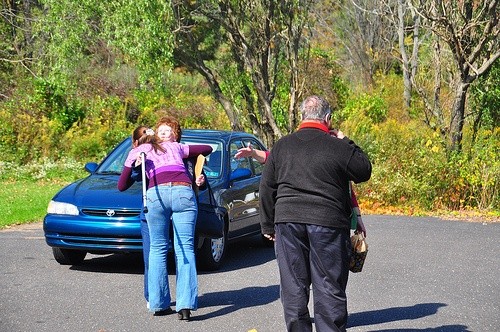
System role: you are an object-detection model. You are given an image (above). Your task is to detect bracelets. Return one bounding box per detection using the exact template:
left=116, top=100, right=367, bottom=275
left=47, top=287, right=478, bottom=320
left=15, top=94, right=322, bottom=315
left=357, top=215, right=361, bottom=217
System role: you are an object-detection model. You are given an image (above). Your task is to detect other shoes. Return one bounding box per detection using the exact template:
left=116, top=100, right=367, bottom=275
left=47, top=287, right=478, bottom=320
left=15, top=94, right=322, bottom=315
left=179, top=309, right=191, bottom=319
left=154, top=309, right=177, bottom=316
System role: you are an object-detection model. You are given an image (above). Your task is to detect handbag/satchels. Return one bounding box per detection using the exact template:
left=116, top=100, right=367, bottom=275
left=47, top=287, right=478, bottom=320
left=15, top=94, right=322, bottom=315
left=194, top=181, right=228, bottom=239
left=349, top=233, right=369, bottom=273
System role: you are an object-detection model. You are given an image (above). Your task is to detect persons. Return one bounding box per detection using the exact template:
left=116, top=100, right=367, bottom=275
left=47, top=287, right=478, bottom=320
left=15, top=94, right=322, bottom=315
left=118, top=125, right=213, bottom=320
left=234, top=94, right=372, bottom=332
left=139, top=117, right=208, bottom=309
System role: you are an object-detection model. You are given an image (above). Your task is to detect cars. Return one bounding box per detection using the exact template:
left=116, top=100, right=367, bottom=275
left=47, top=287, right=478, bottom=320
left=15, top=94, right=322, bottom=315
left=42, top=129, right=274, bottom=271
